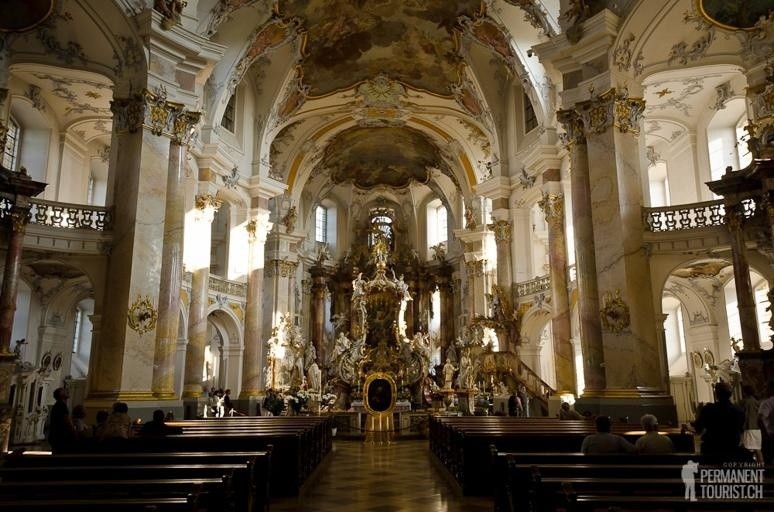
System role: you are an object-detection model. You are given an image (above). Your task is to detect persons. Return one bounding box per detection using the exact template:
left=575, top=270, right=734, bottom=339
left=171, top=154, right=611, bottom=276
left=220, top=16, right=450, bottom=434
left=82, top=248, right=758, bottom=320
left=289, top=332, right=475, bottom=390
left=43, top=380, right=774, bottom=458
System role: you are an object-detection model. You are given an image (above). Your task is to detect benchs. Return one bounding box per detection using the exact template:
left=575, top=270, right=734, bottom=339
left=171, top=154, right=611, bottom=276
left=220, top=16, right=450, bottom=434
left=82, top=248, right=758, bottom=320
left=428, top=408, right=774, bottom=512
left=2, top=411, right=335, bottom=512
